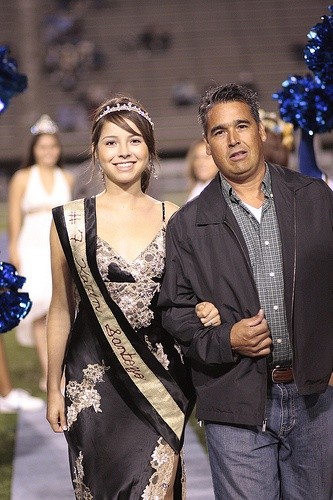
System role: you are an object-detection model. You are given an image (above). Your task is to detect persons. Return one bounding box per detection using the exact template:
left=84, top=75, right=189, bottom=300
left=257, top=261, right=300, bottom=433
left=0, top=335, right=44, bottom=413
left=185, top=139, right=218, bottom=204
left=46, top=97, right=221, bottom=500
left=157, top=83, right=333, bottom=500
left=7, top=114, right=74, bottom=392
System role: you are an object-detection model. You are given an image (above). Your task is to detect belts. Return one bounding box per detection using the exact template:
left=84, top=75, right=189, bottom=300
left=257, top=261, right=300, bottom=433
left=267, top=366, right=294, bottom=383
